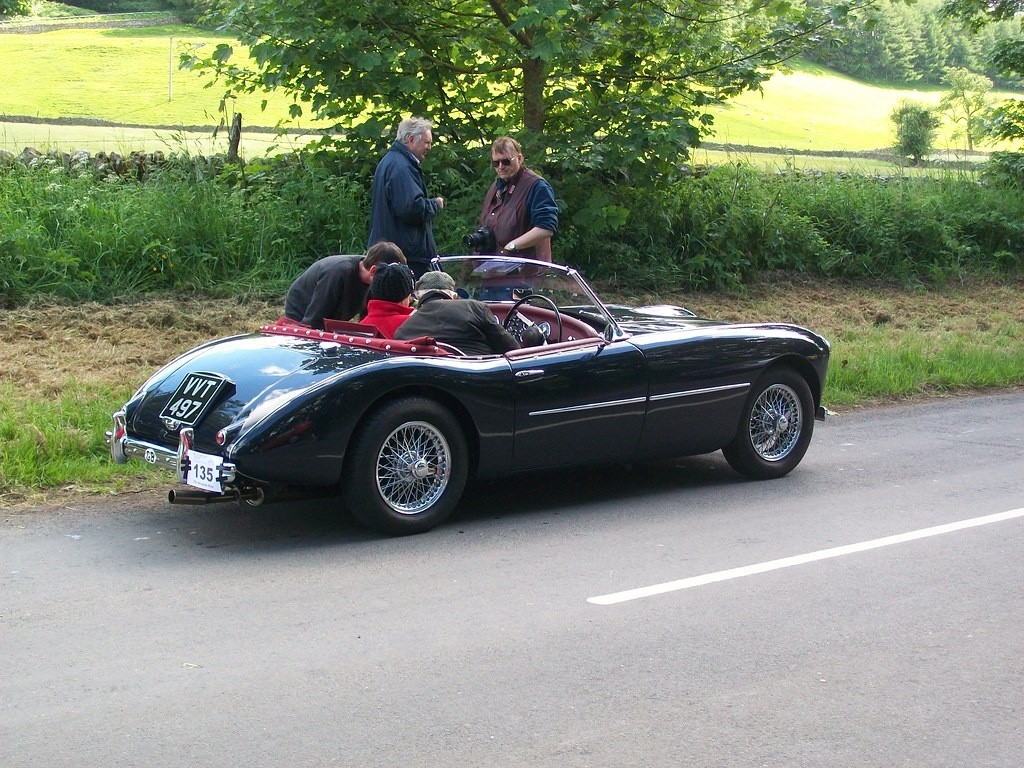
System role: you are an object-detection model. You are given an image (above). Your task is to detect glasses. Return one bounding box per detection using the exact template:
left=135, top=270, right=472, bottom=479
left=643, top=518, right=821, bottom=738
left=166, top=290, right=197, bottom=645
left=492, top=155, right=517, bottom=168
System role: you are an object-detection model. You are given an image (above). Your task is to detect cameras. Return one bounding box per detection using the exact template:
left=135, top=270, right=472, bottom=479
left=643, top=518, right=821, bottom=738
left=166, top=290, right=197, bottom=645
left=463, top=224, right=496, bottom=254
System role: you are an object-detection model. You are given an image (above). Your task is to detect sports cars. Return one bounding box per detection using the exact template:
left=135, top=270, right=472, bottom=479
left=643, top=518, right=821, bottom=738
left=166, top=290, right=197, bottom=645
left=101, top=254, right=832, bottom=536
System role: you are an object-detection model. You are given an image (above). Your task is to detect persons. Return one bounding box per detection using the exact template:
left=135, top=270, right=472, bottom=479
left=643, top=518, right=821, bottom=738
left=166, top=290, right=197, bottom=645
left=367, top=117, right=444, bottom=281
left=358, top=263, right=520, bottom=356
left=285, top=242, right=407, bottom=331
left=472, top=136, right=558, bottom=305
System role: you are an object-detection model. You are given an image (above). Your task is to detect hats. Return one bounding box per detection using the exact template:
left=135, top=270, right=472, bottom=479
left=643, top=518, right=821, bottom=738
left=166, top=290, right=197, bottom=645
left=414, top=270, right=456, bottom=290
left=371, top=262, right=415, bottom=302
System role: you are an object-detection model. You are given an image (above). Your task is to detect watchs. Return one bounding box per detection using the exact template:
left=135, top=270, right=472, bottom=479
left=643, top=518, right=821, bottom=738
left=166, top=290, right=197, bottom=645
left=509, top=242, right=519, bottom=252
